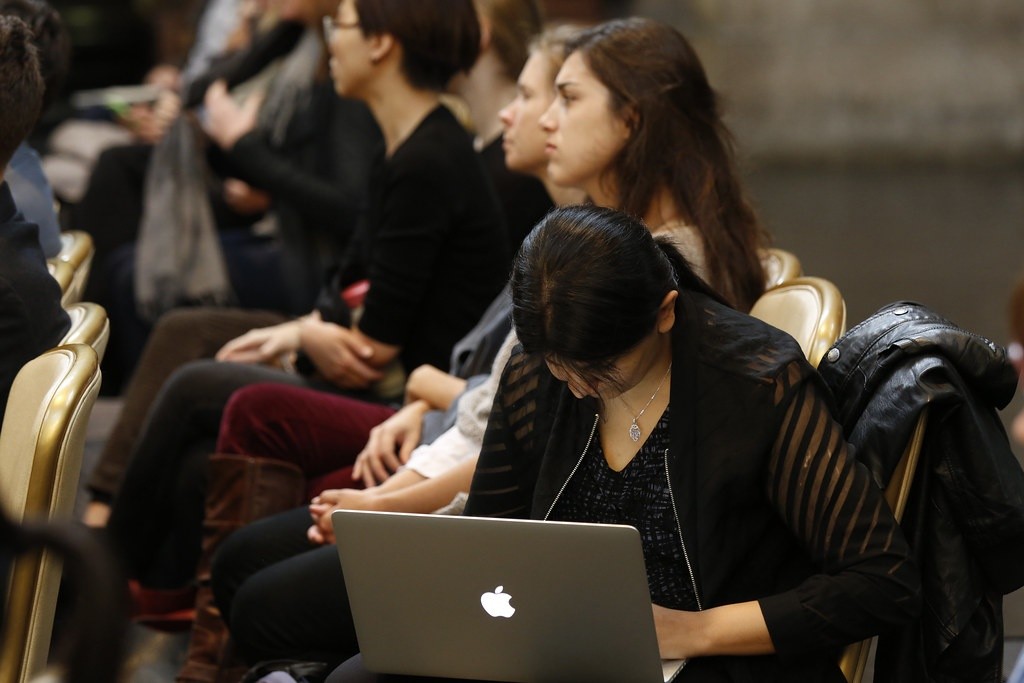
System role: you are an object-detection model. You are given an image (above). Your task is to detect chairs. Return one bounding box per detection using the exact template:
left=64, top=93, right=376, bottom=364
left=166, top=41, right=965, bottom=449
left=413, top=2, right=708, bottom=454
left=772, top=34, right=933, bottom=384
left=0, top=220, right=110, bottom=681
left=736, top=245, right=927, bottom=682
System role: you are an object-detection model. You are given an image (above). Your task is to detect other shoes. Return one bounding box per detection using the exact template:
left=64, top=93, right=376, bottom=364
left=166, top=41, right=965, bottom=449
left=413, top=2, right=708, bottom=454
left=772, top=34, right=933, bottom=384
left=133, top=592, right=199, bottom=624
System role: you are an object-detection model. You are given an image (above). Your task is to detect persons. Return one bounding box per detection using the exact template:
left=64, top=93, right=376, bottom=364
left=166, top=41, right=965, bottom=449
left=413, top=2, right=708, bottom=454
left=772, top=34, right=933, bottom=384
left=325, top=204, right=911, bottom=683
left=0, top=0, right=765, bottom=683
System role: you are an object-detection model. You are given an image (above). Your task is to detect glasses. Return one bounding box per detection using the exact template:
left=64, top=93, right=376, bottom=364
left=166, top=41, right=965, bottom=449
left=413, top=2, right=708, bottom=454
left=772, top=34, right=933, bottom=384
left=322, top=14, right=361, bottom=47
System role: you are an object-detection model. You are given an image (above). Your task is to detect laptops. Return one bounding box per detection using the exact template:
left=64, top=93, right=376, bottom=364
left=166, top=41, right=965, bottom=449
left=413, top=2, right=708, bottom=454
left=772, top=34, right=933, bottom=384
left=331, top=508, right=688, bottom=683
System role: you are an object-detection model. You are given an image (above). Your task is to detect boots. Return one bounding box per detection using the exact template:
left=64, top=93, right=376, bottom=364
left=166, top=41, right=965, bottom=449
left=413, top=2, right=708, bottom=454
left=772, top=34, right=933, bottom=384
left=176, top=454, right=301, bottom=683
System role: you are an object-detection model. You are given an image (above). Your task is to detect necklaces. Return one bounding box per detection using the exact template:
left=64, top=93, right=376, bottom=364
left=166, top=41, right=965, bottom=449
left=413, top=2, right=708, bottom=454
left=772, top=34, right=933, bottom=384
left=626, top=363, right=671, bottom=442
